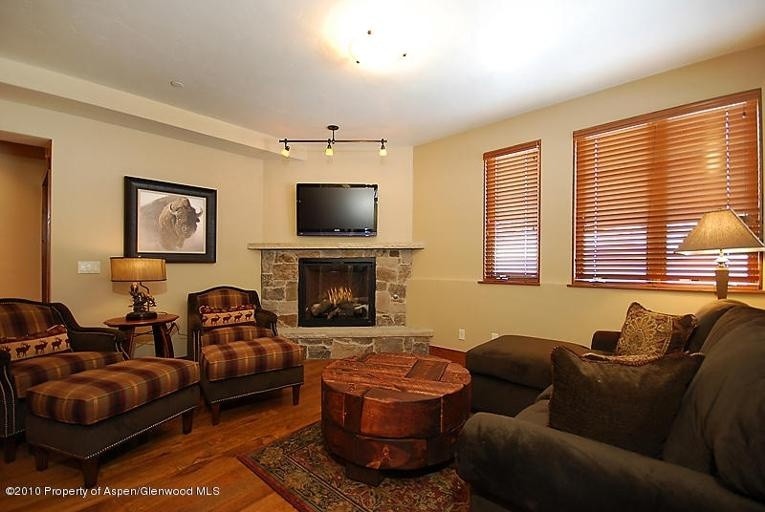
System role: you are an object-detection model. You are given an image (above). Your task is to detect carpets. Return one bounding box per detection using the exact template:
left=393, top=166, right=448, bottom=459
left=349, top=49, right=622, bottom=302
left=237, top=419, right=470, bottom=512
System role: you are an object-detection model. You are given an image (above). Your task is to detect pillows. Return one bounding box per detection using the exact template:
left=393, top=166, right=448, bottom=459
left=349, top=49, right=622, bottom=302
left=614, top=302, right=699, bottom=357
left=549, top=344, right=706, bottom=461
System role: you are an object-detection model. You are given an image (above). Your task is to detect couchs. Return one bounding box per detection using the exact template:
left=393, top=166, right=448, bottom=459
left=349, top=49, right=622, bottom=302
left=456, top=299, right=765, bottom=512
left=187, top=285, right=305, bottom=426
left=0, top=298, right=132, bottom=463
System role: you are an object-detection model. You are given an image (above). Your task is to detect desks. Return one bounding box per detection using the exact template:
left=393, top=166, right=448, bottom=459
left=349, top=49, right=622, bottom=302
left=103, top=312, right=180, bottom=359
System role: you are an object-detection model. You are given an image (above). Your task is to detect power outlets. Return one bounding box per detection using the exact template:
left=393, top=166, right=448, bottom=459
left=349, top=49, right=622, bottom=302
left=76, top=261, right=101, bottom=274
left=458, top=329, right=466, bottom=339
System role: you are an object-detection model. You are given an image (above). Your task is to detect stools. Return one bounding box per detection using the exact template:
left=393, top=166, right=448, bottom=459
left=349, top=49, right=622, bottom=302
left=25, top=356, right=200, bottom=489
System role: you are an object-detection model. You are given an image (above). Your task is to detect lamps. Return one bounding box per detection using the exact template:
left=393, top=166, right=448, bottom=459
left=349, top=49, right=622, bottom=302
left=338, top=10, right=421, bottom=83
left=279, top=124, right=388, bottom=158
left=110, top=256, right=167, bottom=321
left=673, top=208, right=765, bottom=300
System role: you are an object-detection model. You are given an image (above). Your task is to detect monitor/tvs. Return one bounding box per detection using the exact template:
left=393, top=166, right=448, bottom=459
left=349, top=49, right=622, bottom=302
left=296, top=183, right=378, bottom=237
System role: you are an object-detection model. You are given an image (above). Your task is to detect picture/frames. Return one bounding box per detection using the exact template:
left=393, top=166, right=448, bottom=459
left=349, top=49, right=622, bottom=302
left=123, top=175, right=217, bottom=264
